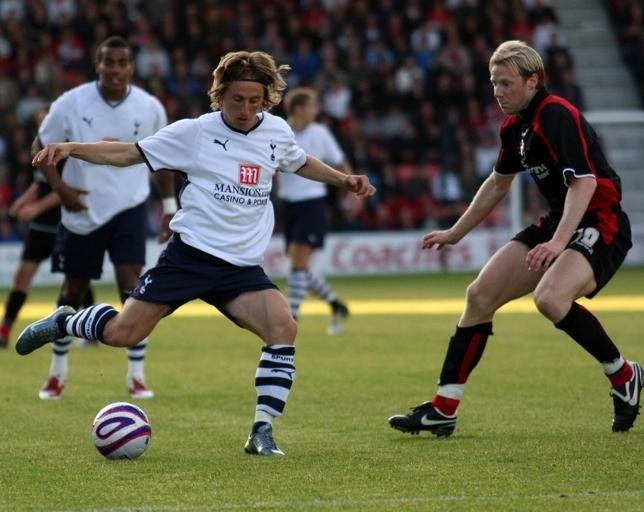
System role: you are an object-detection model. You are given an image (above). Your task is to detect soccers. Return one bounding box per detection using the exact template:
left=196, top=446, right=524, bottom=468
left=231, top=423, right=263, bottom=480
left=91, top=402, right=151, bottom=460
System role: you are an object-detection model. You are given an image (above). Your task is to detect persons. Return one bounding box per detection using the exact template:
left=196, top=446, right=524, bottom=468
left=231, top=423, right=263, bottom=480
left=31, top=37, right=177, bottom=401
left=0, top=106, right=96, bottom=346
left=15, top=50, right=377, bottom=455
left=1, top=4, right=644, bottom=241
left=273, top=87, right=348, bottom=335
left=389, top=40, right=643, bottom=437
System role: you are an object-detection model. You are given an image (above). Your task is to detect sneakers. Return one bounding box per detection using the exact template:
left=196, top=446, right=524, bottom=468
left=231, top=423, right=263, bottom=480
left=611, top=363, right=644, bottom=433
left=389, top=401, right=458, bottom=437
left=40, top=374, right=67, bottom=401
left=326, top=306, right=350, bottom=336
left=14, top=304, right=76, bottom=356
left=245, top=424, right=284, bottom=457
left=126, top=376, right=153, bottom=399
